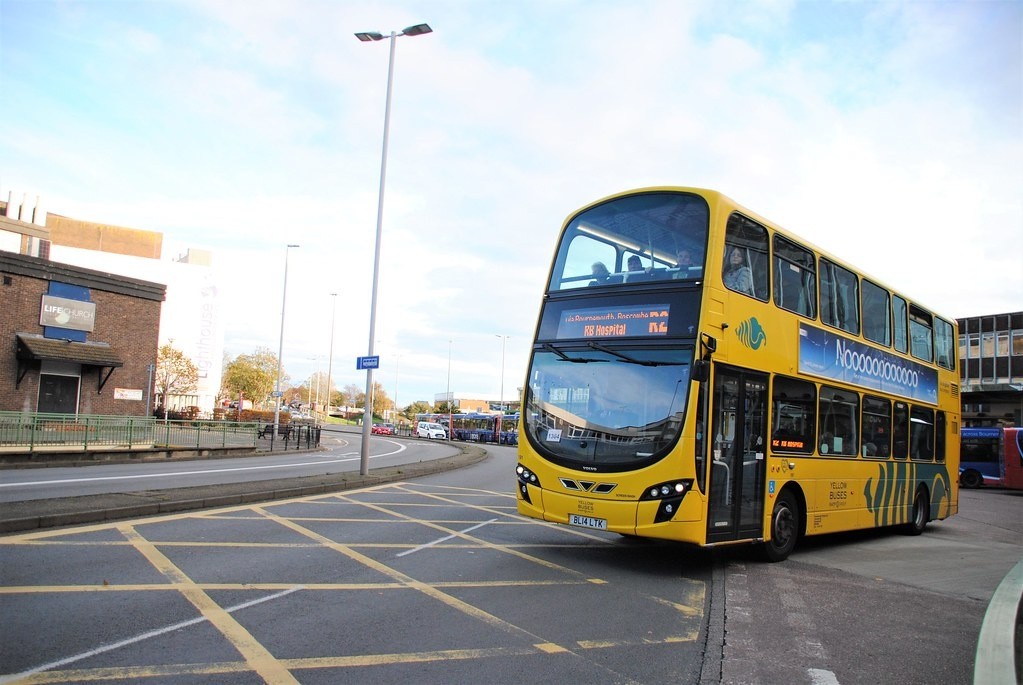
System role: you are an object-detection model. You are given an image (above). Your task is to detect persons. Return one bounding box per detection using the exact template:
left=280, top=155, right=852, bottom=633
left=938, top=356, right=946, bottom=366
left=724, top=245, right=753, bottom=297
left=822, top=428, right=891, bottom=456
left=580, top=249, right=698, bottom=287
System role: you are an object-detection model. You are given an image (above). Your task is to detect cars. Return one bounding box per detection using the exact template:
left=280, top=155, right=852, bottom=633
left=222, top=399, right=239, bottom=408
left=280, top=398, right=308, bottom=420
left=382, top=423, right=399, bottom=435
left=372, top=423, right=391, bottom=435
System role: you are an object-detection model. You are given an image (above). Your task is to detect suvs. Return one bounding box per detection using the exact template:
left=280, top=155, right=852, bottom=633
left=417, top=421, right=447, bottom=440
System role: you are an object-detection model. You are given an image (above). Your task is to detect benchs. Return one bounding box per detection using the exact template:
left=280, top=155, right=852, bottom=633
left=258, top=425, right=292, bottom=441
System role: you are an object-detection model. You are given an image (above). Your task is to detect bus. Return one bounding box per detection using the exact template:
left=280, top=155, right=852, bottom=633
left=958, top=426, right=1023, bottom=491
left=412, top=412, right=538, bottom=446
left=514, top=183, right=962, bottom=565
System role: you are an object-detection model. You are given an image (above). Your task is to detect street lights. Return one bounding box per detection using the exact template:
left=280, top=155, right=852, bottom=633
left=325, top=292, right=342, bottom=417
left=352, top=21, right=436, bottom=478
left=495, top=333, right=510, bottom=446
left=273, top=243, right=302, bottom=442
left=164, top=338, right=177, bottom=425
